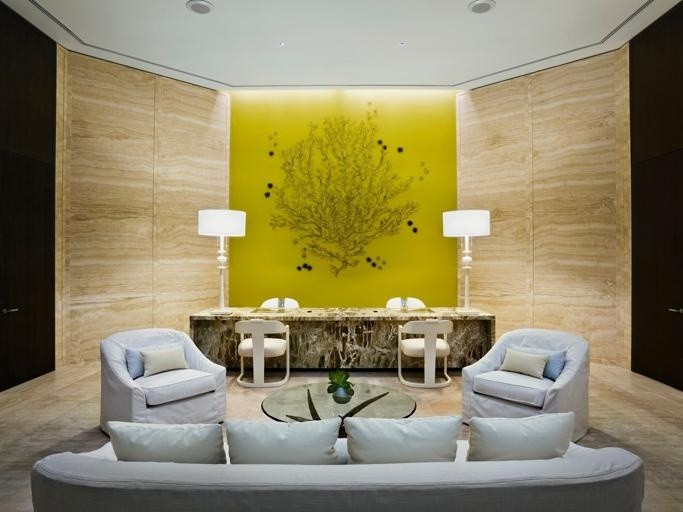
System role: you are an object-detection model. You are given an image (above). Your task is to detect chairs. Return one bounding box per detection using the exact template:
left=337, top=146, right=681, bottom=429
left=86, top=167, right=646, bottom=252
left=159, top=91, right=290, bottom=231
left=260, top=298, right=300, bottom=310
left=386, top=298, right=426, bottom=311
left=398, top=320, right=451, bottom=388
left=235, top=321, right=290, bottom=387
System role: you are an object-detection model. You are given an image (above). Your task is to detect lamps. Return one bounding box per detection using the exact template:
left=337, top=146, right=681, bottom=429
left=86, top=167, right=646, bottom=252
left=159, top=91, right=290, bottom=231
left=443, top=209, right=490, bottom=315
left=198, top=210, right=245, bottom=315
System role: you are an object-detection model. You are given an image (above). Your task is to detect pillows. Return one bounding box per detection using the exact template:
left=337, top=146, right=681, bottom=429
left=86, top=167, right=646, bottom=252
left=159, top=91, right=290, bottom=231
left=124, top=349, right=144, bottom=380
left=467, top=411, right=576, bottom=462
left=498, top=350, right=547, bottom=380
left=106, top=420, right=226, bottom=465
left=229, top=419, right=340, bottom=464
left=343, top=417, right=459, bottom=463
left=141, top=345, right=188, bottom=377
left=509, top=345, right=567, bottom=380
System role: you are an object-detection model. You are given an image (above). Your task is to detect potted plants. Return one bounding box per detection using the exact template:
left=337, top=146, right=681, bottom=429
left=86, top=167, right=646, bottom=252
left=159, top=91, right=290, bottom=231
left=327, top=372, right=354, bottom=403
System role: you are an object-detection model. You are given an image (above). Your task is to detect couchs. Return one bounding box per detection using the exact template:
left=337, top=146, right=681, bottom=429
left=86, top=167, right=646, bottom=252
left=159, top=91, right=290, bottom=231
left=461, top=329, right=590, bottom=442
left=31, top=441, right=643, bottom=512
left=101, top=329, right=227, bottom=435
